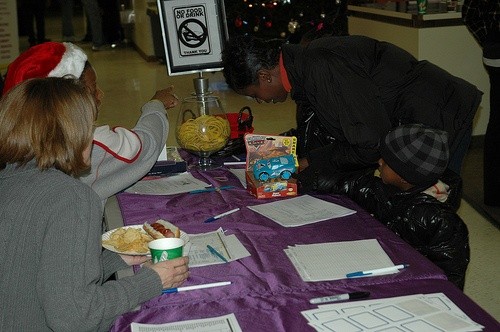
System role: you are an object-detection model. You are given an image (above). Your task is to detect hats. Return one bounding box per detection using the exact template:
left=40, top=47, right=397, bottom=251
left=3, top=42, right=88, bottom=96
left=379, top=123, right=450, bottom=189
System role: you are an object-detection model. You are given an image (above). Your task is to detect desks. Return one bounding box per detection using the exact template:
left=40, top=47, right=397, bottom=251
left=105, top=148, right=499, bottom=332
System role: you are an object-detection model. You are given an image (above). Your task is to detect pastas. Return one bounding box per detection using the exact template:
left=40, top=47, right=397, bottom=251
left=175, top=115, right=231, bottom=151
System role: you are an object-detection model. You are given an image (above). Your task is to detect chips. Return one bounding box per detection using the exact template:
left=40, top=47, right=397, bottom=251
left=101, top=227, right=152, bottom=253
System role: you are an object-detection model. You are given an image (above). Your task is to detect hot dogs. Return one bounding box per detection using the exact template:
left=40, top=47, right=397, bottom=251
left=143, top=220, right=181, bottom=240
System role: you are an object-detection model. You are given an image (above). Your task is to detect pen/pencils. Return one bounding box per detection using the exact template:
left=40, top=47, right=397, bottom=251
left=206, top=244, right=229, bottom=263
left=161, top=281, right=234, bottom=293
left=310, top=291, right=373, bottom=304
left=205, top=206, right=243, bottom=222
left=190, top=186, right=235, bottom=193
left=346, top=263, right=410, bottom=277
left=222, top=162, right=247, bottom=165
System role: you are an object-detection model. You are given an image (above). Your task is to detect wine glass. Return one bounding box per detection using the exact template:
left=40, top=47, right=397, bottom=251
left=175, top=95, right=233, bottom=171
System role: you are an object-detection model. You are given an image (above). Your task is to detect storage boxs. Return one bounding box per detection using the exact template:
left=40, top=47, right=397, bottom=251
left=244, top=133, right=298, bottom=199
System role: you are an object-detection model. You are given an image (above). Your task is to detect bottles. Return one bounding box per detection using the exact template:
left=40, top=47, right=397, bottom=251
left=417, top=0, right=428, bottom=14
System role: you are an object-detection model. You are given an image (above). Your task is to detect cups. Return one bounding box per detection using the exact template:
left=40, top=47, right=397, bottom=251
left=148, top=238, right=184, bottom=264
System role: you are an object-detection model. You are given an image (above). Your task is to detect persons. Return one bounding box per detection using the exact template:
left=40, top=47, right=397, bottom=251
left=295, top=123, right=470, bottom=290
left=29, top=0, right=125, bottom=50
left=222, top=34, right=483, bottom=208
left=463, top=0, right=500, bottom=207
left=0, top=41, right=177, bottom=208
left=0, top=77, right=192, bottom=332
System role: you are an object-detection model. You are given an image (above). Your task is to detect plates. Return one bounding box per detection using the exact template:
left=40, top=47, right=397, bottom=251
left=101, top=225, right=189, bottom=255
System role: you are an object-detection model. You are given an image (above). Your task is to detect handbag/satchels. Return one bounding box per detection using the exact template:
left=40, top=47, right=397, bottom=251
left=182, top=106, right=254, bottom=140
